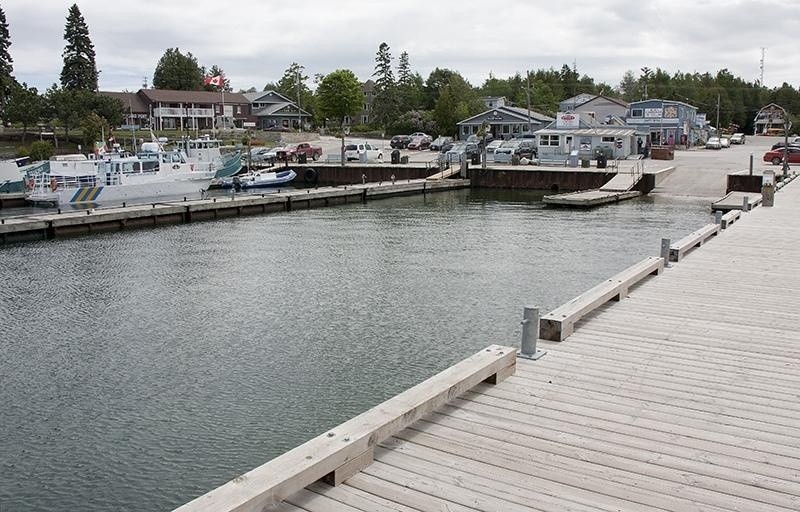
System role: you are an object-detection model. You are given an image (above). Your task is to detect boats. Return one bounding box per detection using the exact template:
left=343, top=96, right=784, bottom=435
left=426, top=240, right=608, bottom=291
left=0, top=97, right=298, bottom=207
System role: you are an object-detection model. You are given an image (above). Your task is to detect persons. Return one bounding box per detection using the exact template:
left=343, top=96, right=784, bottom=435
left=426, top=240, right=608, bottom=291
left=637, top=137, right=643, bottom=153
left=668, top=135, right=673, bottom=144
left=781, top=151, right=792, bottom=170
left=51, top=178, right=58, bottom=192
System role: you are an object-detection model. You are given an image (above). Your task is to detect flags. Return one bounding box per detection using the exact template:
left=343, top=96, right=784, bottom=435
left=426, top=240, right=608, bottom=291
left=204, top=75, right=224, bottom=88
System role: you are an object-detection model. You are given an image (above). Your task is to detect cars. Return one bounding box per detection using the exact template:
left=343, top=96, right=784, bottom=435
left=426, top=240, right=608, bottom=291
left=705, top=133, right=746, bottom=150
left=344, top=143, right=384, bottom=161
left=390, top=132, right=538, bottom=160
left=239, top=142, right=323, bottom=164
left=763, top=136, right=800, bottom=165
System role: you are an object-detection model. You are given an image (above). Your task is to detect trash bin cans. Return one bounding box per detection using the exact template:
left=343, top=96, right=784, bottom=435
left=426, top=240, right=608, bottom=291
left=391, top=150, right=400, bottom=164
left=298, top=152, right=306, bottom=164
left=471, top=149, right=480, bottom=165
left=597, top=152, right=607, bottom=168
